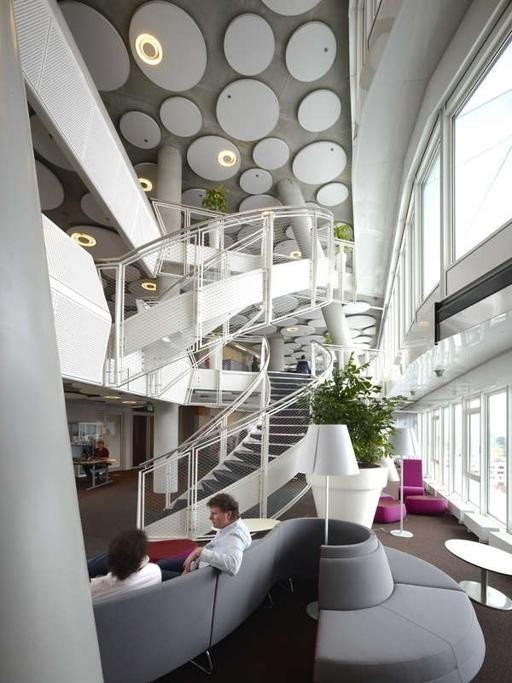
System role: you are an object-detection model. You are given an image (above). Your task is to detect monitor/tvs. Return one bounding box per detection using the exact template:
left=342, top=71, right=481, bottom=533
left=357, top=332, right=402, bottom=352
left=81, top=444, right=95, bottom=458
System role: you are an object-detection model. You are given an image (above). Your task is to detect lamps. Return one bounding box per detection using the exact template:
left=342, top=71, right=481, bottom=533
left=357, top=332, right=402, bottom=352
left=296, top=422, right=360, bottom=545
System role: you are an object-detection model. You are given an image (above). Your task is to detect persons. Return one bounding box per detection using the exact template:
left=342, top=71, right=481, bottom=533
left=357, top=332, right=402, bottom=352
left=89, top=528, right=162, bottom=604
left=156, top=492, right=254, bottom=586
left=82, top=440, right=109, bottom=484
left=295, top=354, right=311, bottom=373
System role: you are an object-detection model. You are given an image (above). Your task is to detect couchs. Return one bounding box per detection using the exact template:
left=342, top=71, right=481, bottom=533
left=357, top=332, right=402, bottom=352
left=94, top=517, right=486, bottom=682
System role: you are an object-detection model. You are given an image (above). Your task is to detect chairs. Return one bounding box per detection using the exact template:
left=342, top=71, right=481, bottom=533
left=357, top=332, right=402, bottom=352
left=399, top=458, right=424, bottom=502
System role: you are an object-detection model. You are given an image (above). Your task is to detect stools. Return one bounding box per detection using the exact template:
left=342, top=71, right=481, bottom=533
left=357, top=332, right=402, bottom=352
left=374, top=495, right=446, bottom=522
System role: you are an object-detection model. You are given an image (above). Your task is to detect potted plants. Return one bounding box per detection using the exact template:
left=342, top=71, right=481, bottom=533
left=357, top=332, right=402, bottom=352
left=294, top=367, right=409, bottom=526
left=200, top=182, right=229, bottom=249
left=335, top=223, right=353, bottom=271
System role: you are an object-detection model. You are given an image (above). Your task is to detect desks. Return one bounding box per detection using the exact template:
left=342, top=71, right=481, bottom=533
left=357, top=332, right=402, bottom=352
left=73, top=458, right=114, bottom=491
